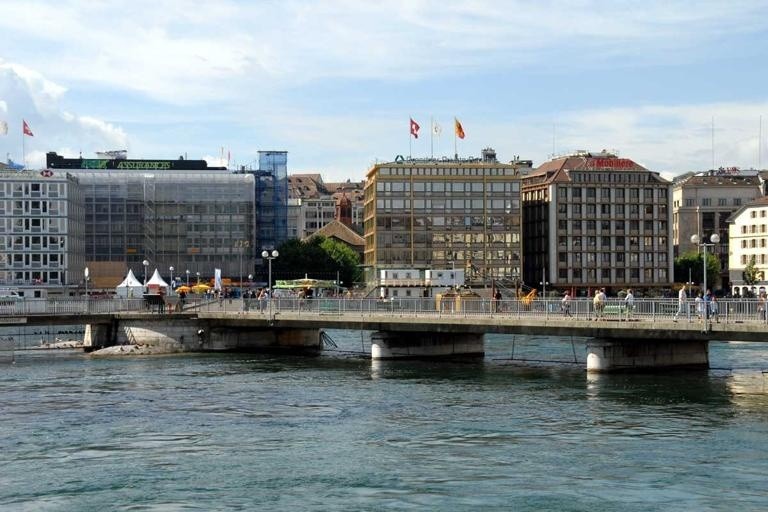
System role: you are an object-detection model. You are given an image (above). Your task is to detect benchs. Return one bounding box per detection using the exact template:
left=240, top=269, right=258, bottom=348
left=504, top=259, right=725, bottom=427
left=662, top=304, right=696, bottom=318
left=377, top=301, right=399, bottom=312
left=604, top=305, right=635, bottom=318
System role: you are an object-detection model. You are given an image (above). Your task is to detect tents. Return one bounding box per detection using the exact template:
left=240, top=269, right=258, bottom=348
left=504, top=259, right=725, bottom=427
left=117, top=268, right=169, bottom=288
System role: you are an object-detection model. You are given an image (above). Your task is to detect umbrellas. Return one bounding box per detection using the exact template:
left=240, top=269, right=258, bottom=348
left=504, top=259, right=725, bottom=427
left=177, top=284, right=210, bottom=294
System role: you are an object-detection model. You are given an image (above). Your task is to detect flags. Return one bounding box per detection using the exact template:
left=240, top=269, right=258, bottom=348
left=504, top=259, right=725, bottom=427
left=410, top=119, right=419, bottom=138
left=23, top=119, right=34, bottom=137
left=455, top=119, right=465, bottom=140
left=432, top=120, right=442, bottom=135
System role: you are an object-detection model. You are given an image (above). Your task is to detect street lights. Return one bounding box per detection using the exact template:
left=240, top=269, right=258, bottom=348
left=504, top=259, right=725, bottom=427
left=447, top=261, right=456, bottom=287
left=539, top=281, right=549, bottom=300
left=261, top=249, right=279, bottom=328
left=84, top=265, right=91, bottom=299
left=689, top=233, right=723, bottom=333
left=332, top=280, right=343, bottom=297
left=686, top=281, right=695, bottom=297
left=247, top=273, right=255, bottom=291
left=142, top=259, right=201, bottom=298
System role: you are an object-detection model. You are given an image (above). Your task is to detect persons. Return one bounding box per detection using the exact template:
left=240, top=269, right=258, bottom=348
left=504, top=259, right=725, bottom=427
left=617, top=288, right=634, bottom=306
left=757, top=292, right=768, bottom=324
left=180, top=290, right=186, bottom=303
left=243, top=287, right=266, bottom=314
left=560, top=290, right=573, bottom=317
left=296, top=288, right=313, bottom=313
left=158, top=288, right=166, bottom=313
left=695, top=289, right=721, bottom=323
left=592, top=288, right=608, bottom=321
left=377, top=296, right=388, bottom=302
left=494, top=289, right=502, bottom=312
left=673, top=285, right=689, bottom=322
